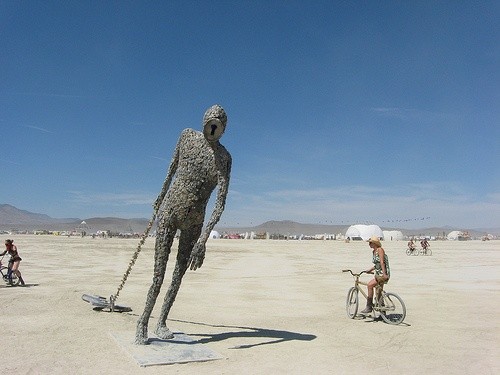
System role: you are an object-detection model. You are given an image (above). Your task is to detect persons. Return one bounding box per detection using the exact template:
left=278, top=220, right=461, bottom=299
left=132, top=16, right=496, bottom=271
left=361, top=237, right=390, bottom=313
left=0, top=239, right=26, bottom=287
left=421, top=238, right=430, bottom=253
left=408, top=239, right=417, bottom=251
left=133, top=102, right=233, bottom=344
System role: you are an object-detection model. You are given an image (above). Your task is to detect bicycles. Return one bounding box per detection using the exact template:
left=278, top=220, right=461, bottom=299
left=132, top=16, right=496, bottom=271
left=0, top=255, right=21, bottom=286
left=405, top=246, right=432, bottom=256
left=342, top=269, right=407, bottom=325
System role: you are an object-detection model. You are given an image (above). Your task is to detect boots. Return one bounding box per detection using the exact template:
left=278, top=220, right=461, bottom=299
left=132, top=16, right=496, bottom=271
left=374, top=305, right=386, bottom=321
left=362, top=297, right=373, bottom=313
left=19, top=276, right=25, bottom=287
left=7, top=277, right=13, bottom=285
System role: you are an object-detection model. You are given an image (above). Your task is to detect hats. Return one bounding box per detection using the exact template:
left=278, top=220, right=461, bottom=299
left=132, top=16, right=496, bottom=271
left=366, top=237, right=381, bottom=247
